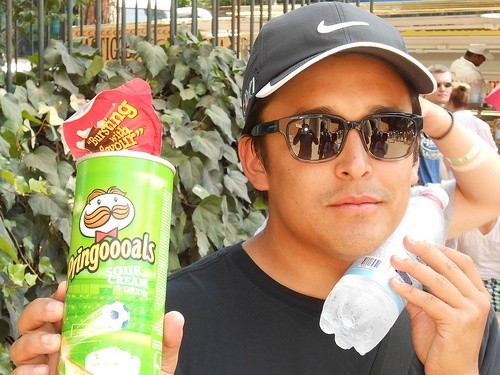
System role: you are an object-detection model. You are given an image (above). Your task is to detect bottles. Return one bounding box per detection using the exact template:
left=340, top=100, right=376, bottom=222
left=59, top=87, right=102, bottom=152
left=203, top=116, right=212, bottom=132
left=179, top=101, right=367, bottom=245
left=318, top=185, right=449, bottom=355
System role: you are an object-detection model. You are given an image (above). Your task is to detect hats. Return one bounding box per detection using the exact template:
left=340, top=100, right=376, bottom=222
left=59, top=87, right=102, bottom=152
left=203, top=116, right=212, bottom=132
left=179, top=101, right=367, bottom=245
left=241, top=3, right=438, bottom=120
left=467, top=43, right=493, bottom=60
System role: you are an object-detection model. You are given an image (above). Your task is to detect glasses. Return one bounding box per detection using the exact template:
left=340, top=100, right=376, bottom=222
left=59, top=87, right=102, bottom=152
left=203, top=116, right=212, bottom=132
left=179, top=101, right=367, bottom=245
left=435, top=82, right=453, bottom=87
left=252, top=112, right=423, bottom=163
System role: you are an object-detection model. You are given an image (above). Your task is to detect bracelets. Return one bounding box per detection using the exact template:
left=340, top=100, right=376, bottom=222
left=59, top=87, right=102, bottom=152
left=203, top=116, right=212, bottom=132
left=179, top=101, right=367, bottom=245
left=455, top=139, right=488, bottom=173
left=448, top=132, right=481, bottom=164
left=427, top=109, right=454, bottom=141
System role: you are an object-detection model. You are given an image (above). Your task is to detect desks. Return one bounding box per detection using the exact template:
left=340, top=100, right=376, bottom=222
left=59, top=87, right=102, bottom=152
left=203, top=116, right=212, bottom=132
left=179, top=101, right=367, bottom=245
left=469, top=110, right=500, bottom=148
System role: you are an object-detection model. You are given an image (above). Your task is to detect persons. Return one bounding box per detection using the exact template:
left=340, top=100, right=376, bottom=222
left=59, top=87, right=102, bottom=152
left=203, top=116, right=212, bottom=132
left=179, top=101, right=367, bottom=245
left=293, top=123, right=317, bottom=160
left=418, top=43, right=500, bottom=325
left=368, top=127, right=414, bottom=158
left=318, top=128, right=344, bottom=159
left=8, top=3, right=500, bottom=375
